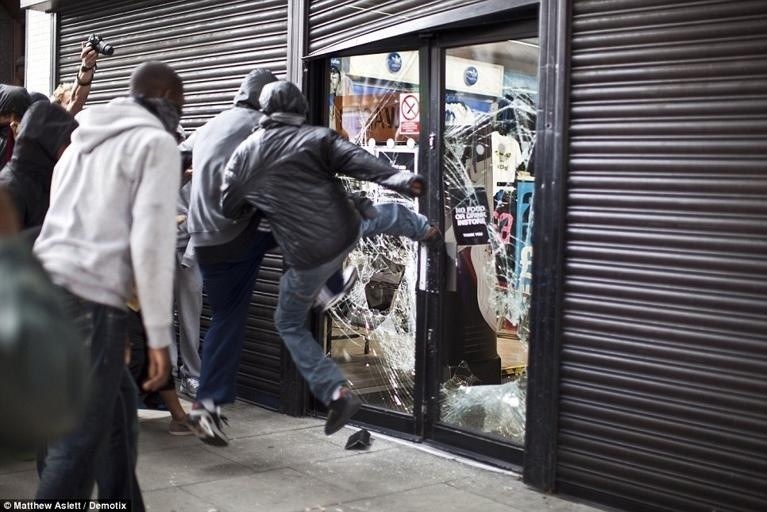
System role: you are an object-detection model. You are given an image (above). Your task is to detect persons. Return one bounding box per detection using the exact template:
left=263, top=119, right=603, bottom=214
left=0, top=41, right=439, bottom=512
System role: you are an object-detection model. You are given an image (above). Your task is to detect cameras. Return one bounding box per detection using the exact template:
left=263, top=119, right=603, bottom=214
left=85, top=34, right=114, bottom=56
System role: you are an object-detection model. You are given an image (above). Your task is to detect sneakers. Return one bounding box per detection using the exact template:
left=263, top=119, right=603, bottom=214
left=420, top=222, right=444, bottom=254
left=178, top=374, right=202, bottom=399
left=168, top=413, right=195, bottom=435
left=184, top=401, right=232, bottom=447
left=322, top=388, right=362, bottom=436
left=314, top=264, right=359, bottom=311
